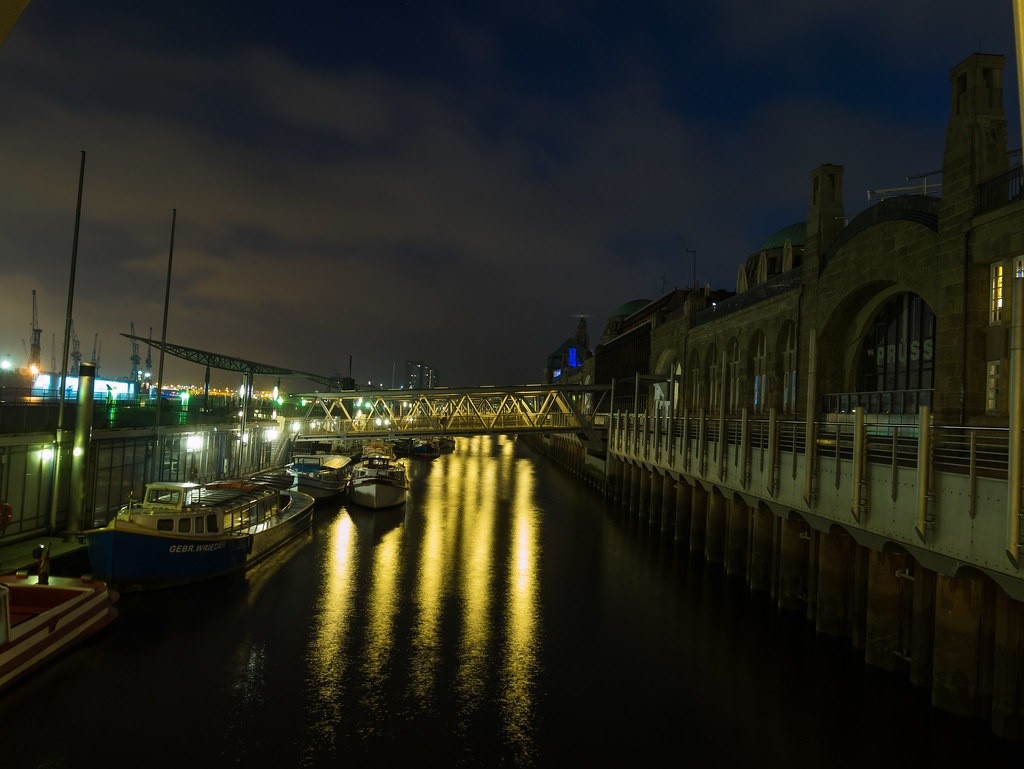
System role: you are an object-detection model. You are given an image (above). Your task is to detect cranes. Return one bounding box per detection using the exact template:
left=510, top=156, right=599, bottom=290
left=30, top=289, right=41, bottom=369
left=145, top=327, right=154, bottom=374
left=70, top=317, right=81, bottom=375
left=130, top=320, right=140, bottom=382
left=89, top=333, right=98, bottom=367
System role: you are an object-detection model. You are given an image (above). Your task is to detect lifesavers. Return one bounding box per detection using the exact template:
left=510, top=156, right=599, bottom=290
left=0, top=503, right=12, bottom=529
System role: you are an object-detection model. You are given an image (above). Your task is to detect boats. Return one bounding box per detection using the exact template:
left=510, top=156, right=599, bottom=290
left=391, top=435, right=424, bottom=453
left=413, top=442, right=440, bottom=458
left=0, top=555, right=120, bottom=705
left=283, top=451, right=353, bottom=502
left=432, top=437, right=456, bottom=450
left=343, top=451, right=410, bottom=509
left=83, top=473, right=315, bottom=596
left=360, top=439, right=398, bottom=461
